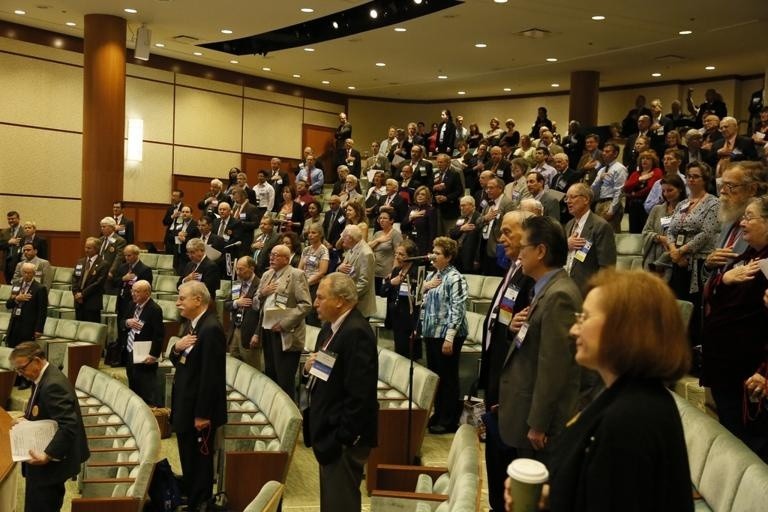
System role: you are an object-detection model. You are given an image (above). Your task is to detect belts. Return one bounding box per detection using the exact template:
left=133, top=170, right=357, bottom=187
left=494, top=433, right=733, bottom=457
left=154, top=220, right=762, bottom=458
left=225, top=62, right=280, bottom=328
left=598, top=197, right=615, bottom=201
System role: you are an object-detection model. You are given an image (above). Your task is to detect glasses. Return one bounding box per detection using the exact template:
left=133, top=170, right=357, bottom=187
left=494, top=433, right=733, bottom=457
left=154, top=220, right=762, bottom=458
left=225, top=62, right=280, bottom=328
left=564, top=194, right=585, bottom=202
left=717, top=181, right=746, bottom=191
left=686, top=174, right=703, bottom=179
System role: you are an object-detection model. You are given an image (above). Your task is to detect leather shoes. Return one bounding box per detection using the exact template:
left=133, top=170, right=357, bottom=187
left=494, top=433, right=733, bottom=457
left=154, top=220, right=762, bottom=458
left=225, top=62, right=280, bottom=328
left=429, top=425, right=448, bottom=434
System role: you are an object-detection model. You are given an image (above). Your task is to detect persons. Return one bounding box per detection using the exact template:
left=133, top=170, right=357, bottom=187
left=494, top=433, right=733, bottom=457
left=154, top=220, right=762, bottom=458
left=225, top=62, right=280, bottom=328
left=1, top=86, right=767, bottom=510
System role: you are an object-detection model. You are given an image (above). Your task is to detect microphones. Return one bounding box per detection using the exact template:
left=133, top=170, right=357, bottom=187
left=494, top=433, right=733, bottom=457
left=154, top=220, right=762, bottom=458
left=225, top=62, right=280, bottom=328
left=404, top=254, right=438, bottom=264
left=225, top=240, right=242, bottom=249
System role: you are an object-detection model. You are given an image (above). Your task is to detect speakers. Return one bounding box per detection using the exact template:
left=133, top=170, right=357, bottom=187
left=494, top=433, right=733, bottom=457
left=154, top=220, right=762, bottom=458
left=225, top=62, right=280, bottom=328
left=133, top=27, right=152, bottom=61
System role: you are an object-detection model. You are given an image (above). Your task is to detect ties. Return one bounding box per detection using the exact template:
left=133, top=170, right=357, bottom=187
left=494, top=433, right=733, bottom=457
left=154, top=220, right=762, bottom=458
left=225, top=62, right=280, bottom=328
left=485, top=264, right=515, bottom=351
left=10, top=218, right=33, bottom=294
left=567, top=224, right=578, bottom=275
left=308, top=169, right=312, bottom=186
left=85, top=218, right=118, bottom=267
left=726, top=224, right=739, bottom=248
left=127, top=306, right=140, bottom=352
left=172, top=206, right=241, bottom=242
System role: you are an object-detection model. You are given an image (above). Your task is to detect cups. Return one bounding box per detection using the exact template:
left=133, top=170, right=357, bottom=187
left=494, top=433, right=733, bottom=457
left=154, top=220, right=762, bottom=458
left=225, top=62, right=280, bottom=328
left=505, top=458, right=550, bottom=511
